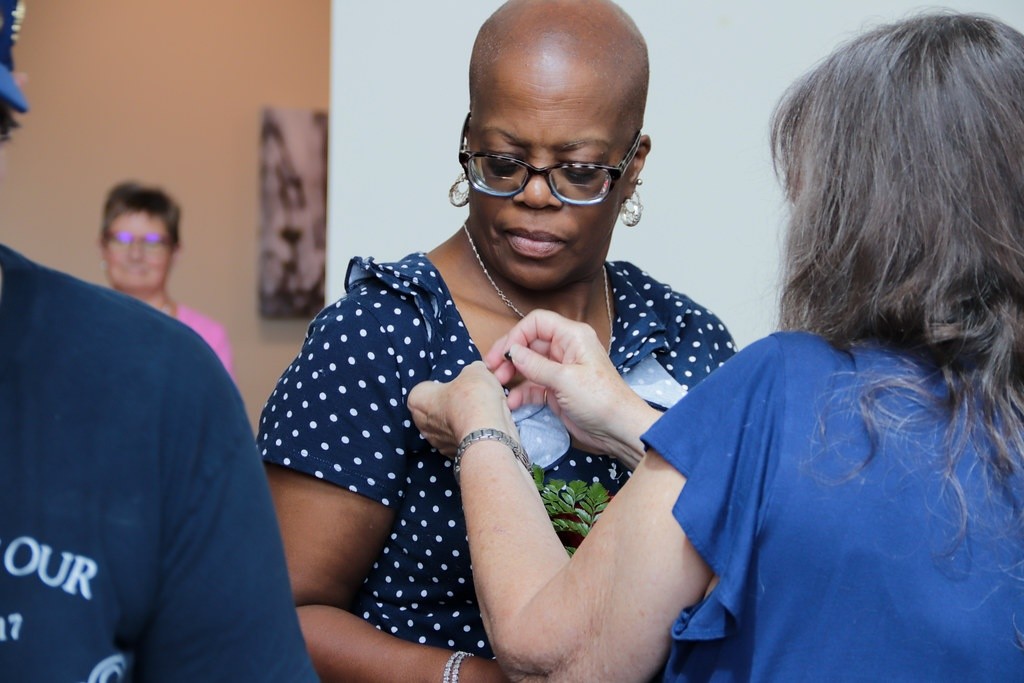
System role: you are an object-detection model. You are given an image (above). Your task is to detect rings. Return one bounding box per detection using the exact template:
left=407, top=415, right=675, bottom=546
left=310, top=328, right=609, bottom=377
left=543, top=388, right=547, bottom=405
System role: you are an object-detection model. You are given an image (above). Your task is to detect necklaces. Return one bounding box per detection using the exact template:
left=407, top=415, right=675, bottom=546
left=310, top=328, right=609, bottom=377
left=463, top=222, right=614, bottom=357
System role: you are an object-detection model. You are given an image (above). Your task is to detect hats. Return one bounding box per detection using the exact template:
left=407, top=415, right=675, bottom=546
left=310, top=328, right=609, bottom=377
left=0, top=0, right=29, bottom=113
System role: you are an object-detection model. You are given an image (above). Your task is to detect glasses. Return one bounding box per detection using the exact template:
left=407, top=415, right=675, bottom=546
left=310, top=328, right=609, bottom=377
left=107, top=232, right=172, bottom=246
left=459, top=112, right=641, bottom=205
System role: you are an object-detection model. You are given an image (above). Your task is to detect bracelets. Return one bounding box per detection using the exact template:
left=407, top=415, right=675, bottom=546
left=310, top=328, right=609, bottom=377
left=453, top=429, right=535, bottom=484
left=443, top=650, right=473, bottom=683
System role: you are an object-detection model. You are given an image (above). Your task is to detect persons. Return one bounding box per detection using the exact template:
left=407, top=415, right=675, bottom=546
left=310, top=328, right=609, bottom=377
left=258, top=0, right=736, bottom=683
left=100, top=183, right=229, bottom=370
left=408, top=17, right=1024, bottom=683
left=0, top=0, right=315, bottom=683
